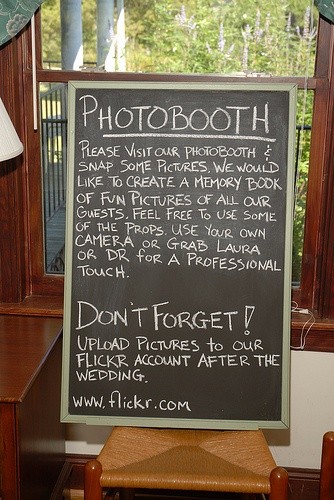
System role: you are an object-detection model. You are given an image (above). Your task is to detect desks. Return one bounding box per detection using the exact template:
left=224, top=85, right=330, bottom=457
left=0, top=315, right=65, bottom=500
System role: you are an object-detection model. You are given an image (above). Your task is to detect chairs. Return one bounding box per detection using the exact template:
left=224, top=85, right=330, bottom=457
left=83, top=426, right=289, bottom=500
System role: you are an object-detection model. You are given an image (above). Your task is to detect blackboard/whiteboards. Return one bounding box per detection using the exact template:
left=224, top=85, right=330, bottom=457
left=59, top=80, right=291, bottom=430
